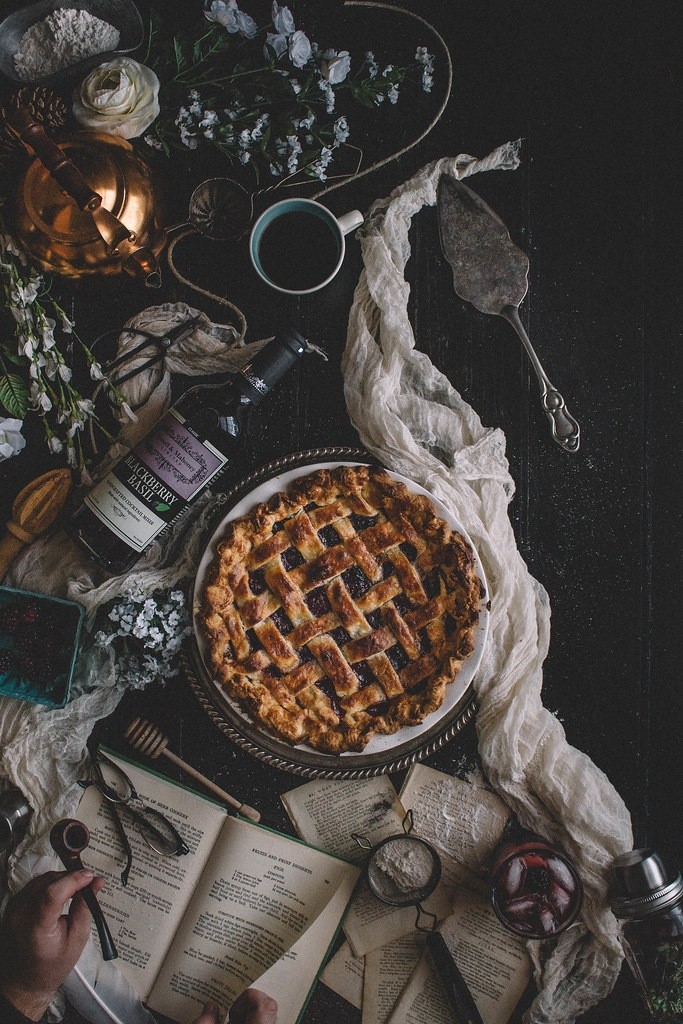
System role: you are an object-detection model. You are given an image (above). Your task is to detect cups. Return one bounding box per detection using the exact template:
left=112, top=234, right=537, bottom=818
left=486, top=826, right=582, bottom=940
left=249, top=198, right=364, bottom=296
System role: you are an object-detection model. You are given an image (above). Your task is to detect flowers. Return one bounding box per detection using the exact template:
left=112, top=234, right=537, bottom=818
left=0, top=229, right=137, bottom=485
left=149, top=0, right=435, bottom=184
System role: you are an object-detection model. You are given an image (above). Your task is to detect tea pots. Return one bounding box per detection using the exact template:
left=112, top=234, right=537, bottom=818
left=1, top=103, right=167, bottom=289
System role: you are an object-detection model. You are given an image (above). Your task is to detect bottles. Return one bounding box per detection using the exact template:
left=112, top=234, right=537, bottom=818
left=607, top=848, right=683, bottom=1024
left=0, top=788, right=32, bottom=854
left=65, top=325, right=309, bottom=576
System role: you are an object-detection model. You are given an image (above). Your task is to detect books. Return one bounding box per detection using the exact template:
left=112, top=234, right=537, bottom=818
left=55, top=744, right=366, bottom=1024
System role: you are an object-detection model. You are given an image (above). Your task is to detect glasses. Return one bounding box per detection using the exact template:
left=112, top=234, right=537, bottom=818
left=76, top=750, right=189, bottom=886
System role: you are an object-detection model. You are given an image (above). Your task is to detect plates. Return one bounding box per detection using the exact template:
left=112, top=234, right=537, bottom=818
left=192, top=462, right=491, bottom=756
left=0, top=0, right=145, bottom=85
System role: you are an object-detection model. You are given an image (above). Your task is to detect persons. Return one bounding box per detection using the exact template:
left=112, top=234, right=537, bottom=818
left=0, top=869, right=278, bottom=1024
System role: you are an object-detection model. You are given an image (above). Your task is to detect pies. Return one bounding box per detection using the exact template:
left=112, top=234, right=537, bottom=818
left=203, top=463, right=490, bottom=756
